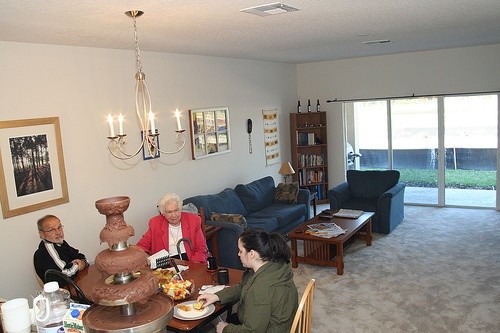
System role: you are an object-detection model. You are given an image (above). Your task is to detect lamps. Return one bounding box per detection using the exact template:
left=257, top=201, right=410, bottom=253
left=106, top=11, right=186, bottom=160
left=279, top=162, right=295, bottom=184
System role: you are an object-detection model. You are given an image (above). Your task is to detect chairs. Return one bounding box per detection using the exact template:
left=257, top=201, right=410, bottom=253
left=44, top=269, right=89, bottom=305
left=290, top=278, right=316, bottom=333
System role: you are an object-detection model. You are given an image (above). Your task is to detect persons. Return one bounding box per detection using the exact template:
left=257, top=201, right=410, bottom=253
left=197, top=228, right=303, bottom=333
left=136, top=193, right=210, bottom=267
left=34, top=215, right=93, bottom=306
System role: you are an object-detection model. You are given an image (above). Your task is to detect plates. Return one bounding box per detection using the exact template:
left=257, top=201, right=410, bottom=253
left=173, top=300, right=215, bottom=320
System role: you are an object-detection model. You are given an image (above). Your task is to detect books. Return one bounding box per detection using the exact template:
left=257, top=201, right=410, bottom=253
left=296, top=131, right=327, bottom=200
left=306, top=209, right=364, bottom=238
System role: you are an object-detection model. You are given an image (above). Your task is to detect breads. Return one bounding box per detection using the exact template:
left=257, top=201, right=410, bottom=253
left=194, top=301, right=204, bottom=309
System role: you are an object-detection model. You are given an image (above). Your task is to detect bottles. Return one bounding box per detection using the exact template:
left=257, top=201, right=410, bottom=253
left=297, top=100, right=301, bottom=113
left=317, top=99, right=320, bottom=112
left=33, top=282, right=74, bottom=333
left=307, top=99, right=311, bottom=112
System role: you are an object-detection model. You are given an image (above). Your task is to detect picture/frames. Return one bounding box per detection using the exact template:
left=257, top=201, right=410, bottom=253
left=189, top=107, right=231, bottom=160
left=141, top=129, right=160, bottom=160
left=0, top=117, right=68, bottom=219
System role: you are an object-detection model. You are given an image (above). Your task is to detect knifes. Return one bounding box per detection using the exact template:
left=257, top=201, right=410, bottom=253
left=171, top=258, right=184, bottom=281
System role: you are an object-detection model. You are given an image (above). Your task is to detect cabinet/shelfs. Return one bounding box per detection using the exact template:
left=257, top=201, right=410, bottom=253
left=290, top=111, right=328, bottom=205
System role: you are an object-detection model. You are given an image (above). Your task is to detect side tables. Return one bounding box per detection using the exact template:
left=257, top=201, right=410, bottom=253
left=309, top=191, right=318, bottom=217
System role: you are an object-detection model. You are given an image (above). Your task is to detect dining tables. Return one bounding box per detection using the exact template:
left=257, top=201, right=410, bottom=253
left=63, top=258, right=244, bottom=333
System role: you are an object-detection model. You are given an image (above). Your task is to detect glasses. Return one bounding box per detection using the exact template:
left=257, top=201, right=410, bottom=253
left=39, top=225, right=64, bottom=233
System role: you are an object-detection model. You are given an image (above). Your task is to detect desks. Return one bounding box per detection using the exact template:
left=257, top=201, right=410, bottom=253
left=288, top=210, right=375, bottom=274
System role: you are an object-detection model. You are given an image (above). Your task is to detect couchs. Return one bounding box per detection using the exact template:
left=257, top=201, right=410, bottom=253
left=182, top=176, right=310, bottom=270
left=328, top=169, right=406, bottom=235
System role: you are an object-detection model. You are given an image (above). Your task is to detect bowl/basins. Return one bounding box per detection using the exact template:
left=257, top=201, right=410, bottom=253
left=162, top=278, right=195, bottom=300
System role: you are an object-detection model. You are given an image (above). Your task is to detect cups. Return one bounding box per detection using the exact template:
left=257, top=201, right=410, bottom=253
left=218, top=269, right=229, bottom=284
left=207, top=257, right=216, bottom=269
left=1, top=298, right=31, bottom=333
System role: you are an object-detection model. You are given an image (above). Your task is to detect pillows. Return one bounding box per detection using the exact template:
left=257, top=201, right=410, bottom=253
left=209, top=212, right=247, bottom=230
left=273, top=182, right=299, bottom=204
